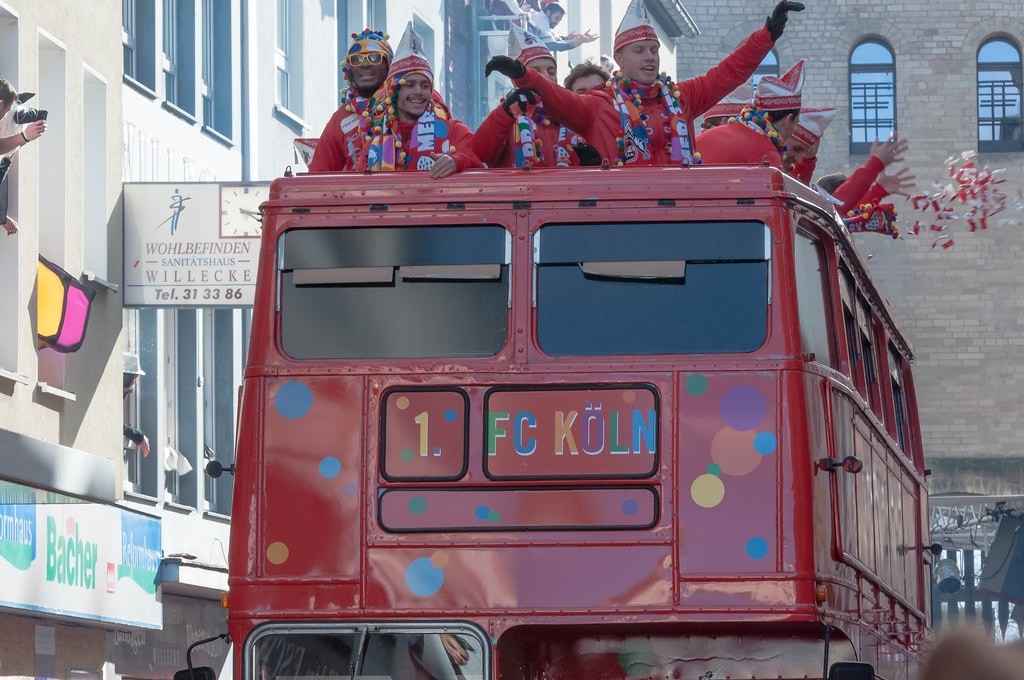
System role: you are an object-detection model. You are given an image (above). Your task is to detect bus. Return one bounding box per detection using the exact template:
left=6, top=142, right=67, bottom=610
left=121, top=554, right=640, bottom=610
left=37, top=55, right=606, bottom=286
left=169, top=162, right=943, bottom=680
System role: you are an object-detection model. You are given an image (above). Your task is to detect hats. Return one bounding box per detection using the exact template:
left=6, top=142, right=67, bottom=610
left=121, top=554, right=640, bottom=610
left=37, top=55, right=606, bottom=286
left=753, top=59, right=805, bottom=111
left=790, top=107, right=837, bottom=146
left=613, top=0, right=664, bottom=52
left=349, top=31, right=393, bottom=63
left=393, top=69, right=434, bottom=91
left=702, top=74, right=754, bottom=121
left=386, top=21, right=433, bottom=77
left=506, top=23, right=557, bottom=66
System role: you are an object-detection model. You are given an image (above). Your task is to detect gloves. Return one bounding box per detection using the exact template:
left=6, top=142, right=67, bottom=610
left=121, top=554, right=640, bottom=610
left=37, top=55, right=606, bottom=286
left=485, top=54, right=528, bottom=79
left=571, top=142, right=601, bottom=165
left=503, top=87, right=543, bottom=112
left=766, top=0, right=805, bottom=41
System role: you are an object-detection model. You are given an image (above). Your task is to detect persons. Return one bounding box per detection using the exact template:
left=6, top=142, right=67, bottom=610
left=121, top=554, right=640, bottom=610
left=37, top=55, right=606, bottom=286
left=0, top=77, right=48, bottom=233
left=921, top=634, right=1024, bottom=680
left=309, top=34, right=445, bottom=173
left=486, top=0, right=600, bottom=52
left=470, top=22, right=603, bottom=166
left=818, top=132, right=915, bottom=220
left=703, top=74, right=754, bottom=130
left=123, top=352, right=150, bottom=456
left=485, top=0, right=807, bottom=165
left=787, top=108, right=839, bottom=184
left=441, top=634, right=475, bottom=680
left=356, top=21, right=484, bottom=179
left=695, top=60, right=806, bottom=171
left=562, top=54, right=613, bottom=95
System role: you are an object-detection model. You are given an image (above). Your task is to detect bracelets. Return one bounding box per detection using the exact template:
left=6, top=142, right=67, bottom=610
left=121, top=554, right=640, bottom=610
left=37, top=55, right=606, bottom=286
left=23, top=132, right=27, bottom=139
left=21, top=132, right=29, bottom=143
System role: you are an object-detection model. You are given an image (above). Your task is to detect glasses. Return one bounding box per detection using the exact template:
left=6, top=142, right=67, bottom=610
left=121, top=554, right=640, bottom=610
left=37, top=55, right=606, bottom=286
left=348, top=53, right=389, bottom=67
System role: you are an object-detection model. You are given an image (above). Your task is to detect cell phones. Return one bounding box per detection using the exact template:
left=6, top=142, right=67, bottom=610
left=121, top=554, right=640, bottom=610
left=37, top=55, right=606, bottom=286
left=829, top=661, right=875, bottom=680
left=38, top=110, right=47, bottom=121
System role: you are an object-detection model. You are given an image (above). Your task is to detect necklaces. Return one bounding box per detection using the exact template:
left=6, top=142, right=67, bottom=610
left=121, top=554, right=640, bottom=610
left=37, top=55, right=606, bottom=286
left=845, top=204, right=899, bottom=238
left=703, top=106, right=786, bottom=161
left=500, top=91, right=573, bottom=166
left=607, top=70, right=702, bottom=166
